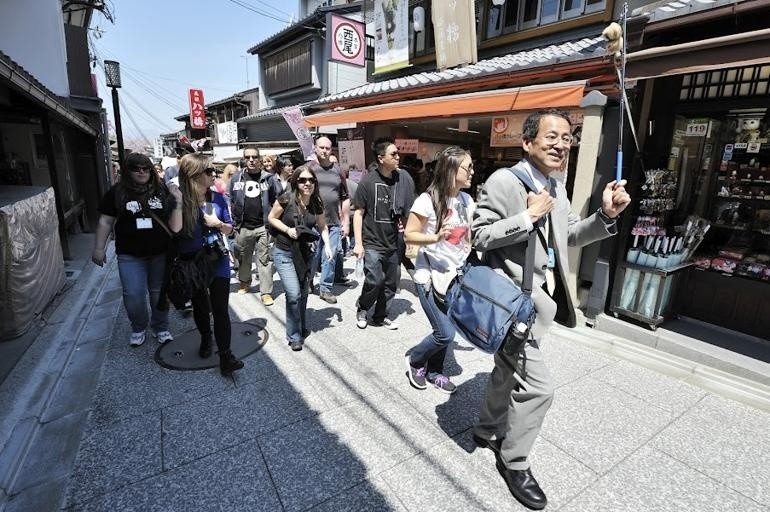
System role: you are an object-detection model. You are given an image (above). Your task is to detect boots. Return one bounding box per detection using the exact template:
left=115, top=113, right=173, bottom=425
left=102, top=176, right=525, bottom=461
left=198, top=332, right=212, bottom=356
left=218, top=350, right=244, bottom=376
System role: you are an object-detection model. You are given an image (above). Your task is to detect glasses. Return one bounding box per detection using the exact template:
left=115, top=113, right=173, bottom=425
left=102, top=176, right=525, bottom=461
left=244, top=155, right=257, bottom=160
left=294, top=176, right=316, bottom=184
left=196, top=166, right=215, bottom=176
left=531, top=133, right=574, bottom=145
left=460, top=163, right=475, bottom=172
left=128, top=163, right=152, bottom=173
left=381, top=151, right=401, bottom=158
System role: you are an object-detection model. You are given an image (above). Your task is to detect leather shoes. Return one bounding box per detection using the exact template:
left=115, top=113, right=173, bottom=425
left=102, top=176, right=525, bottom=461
left=471, top=431, right=502, bottom=452
left=495, top=453, right=548, bottom=510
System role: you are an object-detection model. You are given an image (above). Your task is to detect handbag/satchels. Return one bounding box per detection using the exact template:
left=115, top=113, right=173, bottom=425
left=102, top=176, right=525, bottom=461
left=445, top=254, right=536, bottom=357
left=166, top=250, right=214, bottom=306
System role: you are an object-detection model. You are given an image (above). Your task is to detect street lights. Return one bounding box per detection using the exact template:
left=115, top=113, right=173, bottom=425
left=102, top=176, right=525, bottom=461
left=103, top=59, right=134, bottom=166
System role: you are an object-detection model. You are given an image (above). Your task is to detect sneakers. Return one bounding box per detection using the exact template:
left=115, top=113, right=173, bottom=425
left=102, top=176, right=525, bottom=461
left=238, top=282, right=248, bottom=294
left=151, top=330, right=174, bottom=343
left=373, top=317, right=398, bottom=329
left=356, top=303, right=369, bottom=329
left=335, top=277, right=359, bottom=289
left=260, top=293, right=273, bottom=305
left=428, top=372, right=458, bottom=395
left=130, top=330, right=147, bottom=346
left=290, top=331, right=311, bottom=351
left=405, top=350, right=427, bottom=390
left=319, top=289, right=337, bottom=304
